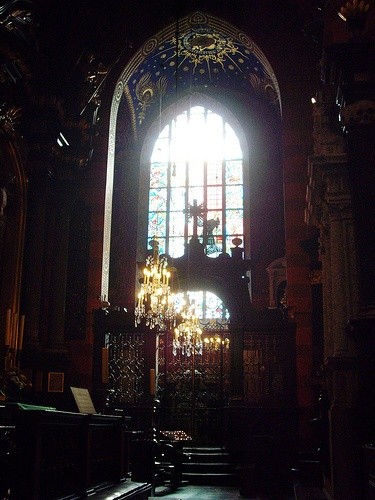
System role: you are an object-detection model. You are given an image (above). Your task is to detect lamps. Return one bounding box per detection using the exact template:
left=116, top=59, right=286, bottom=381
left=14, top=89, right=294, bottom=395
left=134, top=212, right=230, bottom=357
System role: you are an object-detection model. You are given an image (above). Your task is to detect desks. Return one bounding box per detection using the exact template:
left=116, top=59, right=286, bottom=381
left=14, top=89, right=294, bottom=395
left=129, top=440, right=190, bottom=492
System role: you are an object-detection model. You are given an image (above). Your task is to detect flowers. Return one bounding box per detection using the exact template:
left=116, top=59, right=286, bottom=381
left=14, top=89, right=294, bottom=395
left=340, top=0, right=370, bottom=17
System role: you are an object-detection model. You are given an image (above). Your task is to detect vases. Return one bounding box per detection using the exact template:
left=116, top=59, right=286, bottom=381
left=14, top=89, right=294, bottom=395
left=345, top=16, right=366, bottom=32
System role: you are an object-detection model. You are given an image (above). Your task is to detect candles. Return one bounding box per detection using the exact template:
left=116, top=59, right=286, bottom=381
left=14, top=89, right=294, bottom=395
left=19, top=315, right=25, bottom=350
left=10, top=313, right=19, bottom=352
left=5, top=309, right=11, bottom=346
left=150, top=369, right=155, bottom=395
left=102, top=348, right=108, bottom=380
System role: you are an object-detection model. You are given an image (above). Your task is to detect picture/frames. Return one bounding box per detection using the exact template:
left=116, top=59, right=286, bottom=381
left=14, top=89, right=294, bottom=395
left=48, top=372, right=64, bottom=392
